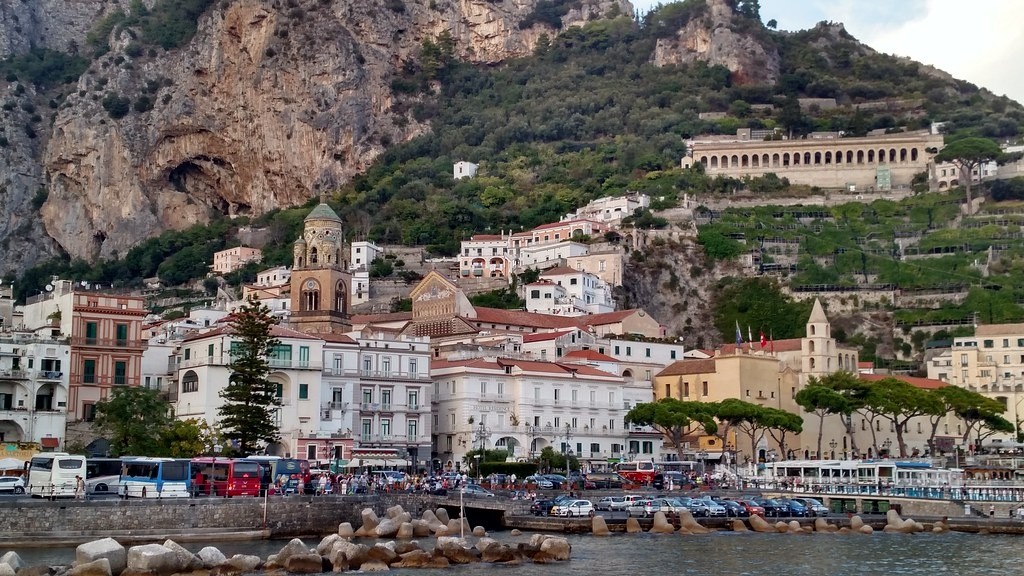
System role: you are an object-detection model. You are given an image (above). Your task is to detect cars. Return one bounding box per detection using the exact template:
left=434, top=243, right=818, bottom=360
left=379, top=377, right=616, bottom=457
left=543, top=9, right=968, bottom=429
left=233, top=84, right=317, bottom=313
left=419, top=460, right=830, bottom=517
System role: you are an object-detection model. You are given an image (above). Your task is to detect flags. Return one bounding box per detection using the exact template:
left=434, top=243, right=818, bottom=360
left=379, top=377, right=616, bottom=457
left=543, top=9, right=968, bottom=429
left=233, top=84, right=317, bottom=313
left=736, top=321, right=774, bottom=353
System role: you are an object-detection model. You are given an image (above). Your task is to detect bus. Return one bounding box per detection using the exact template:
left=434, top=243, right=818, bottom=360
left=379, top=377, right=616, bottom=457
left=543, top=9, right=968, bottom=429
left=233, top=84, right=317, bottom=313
left=23, top=451, right=87, bottom=497
left=86, top=457, right=122, bottom=495
left=118, top=456, right=192, bottom=499
left=190, top=457, right=261, bottom=497
left=247, top=453, right=312, bottom=496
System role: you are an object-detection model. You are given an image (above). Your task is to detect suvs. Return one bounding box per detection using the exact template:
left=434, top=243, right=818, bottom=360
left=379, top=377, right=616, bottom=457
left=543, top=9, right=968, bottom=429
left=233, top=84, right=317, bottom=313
left=0, top=475, right=26, bottom=494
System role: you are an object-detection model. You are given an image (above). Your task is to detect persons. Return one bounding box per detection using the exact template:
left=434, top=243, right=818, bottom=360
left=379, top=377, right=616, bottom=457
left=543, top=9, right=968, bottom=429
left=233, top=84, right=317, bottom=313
left=878, top=478, right=1013, bottom=520
left=2, top=470, right=86, bottom=500
left=604, top=474, right=801, bottom=492
left=274, top=472, right=536, bottom=501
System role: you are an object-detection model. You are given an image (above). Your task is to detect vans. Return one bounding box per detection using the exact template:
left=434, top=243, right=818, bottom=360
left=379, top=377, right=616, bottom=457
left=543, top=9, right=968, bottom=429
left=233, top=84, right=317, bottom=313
left=310, top=469, right=336, bottom=492
left=371, top=470, right=403, bottom=492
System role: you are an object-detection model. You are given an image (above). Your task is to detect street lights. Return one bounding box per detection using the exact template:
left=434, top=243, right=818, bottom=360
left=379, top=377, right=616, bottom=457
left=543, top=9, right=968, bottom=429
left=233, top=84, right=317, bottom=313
left=883, top=438, right=892, bottom=459
left=474, top=422, right=493, bottom=463
left=558, top=426, right=574, bottom=500
left=829, top=439, right=838, bottom=459
left=698, top=448, right=710, bottom=482
left=529, top=424, right=542, bottom=477
left=205, top=437, right=224, bottom=497
left=725, top=441, right=734, bottom=472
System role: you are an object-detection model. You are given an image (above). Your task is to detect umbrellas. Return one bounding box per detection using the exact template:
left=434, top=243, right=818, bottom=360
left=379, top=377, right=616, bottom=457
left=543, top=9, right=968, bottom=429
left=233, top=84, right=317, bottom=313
left=984, top=440, right=1024, bottom=449
